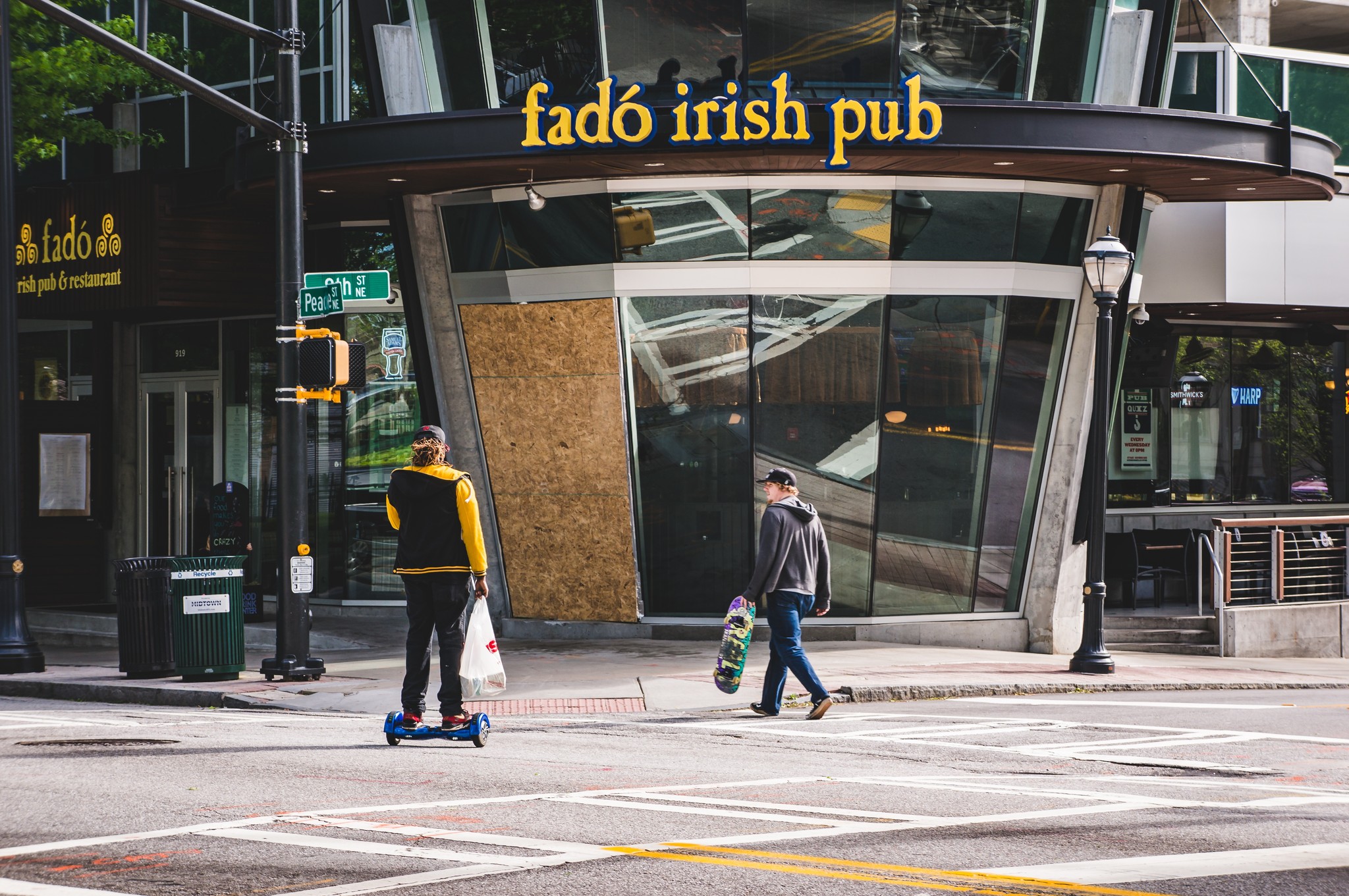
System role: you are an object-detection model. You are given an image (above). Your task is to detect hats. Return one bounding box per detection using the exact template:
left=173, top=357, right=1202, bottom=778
left=414, top=425, right=450, bottom=453
left=756, top=467, right=797, bottom=487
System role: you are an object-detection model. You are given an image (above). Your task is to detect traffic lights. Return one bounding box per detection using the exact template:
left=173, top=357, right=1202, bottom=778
left=347, top=341, right=366, bottom=388
left=298, top=337, right=348, bottom=390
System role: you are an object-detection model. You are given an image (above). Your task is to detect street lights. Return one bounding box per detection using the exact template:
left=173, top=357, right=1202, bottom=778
left=1067, top=225, right=1138, bottom=676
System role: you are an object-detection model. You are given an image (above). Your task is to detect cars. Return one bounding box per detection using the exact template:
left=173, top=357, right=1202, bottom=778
left=345, top=462, right=415, bottom=536
left=1290, top=475, right=1332, bottom=503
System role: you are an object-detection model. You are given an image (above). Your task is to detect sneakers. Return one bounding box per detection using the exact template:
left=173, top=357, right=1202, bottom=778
left=751, top=702, right=771, bottom=715
left=402, top=712, right=424, bottom=730
left=441, top=709, right=474, bottom=731
left=804, top=697, right=832, bottom=720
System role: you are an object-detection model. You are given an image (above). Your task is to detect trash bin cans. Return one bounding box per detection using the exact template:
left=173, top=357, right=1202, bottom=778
left=108, top=556, right=181, bottom=679
left=165, top=555, right=248, bottom=681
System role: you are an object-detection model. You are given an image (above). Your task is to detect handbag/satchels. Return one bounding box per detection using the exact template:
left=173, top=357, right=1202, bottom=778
left=458, top=595, right=506, bottom=698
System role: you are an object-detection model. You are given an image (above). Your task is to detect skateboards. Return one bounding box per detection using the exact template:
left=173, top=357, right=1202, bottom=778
left=713, top=595, right=757, bottom=695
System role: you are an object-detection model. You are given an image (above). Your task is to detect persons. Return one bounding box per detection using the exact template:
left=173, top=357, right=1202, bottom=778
left=385, top=423, right=489, bottom=731
left=739, top=467, right=834, bottom=720
